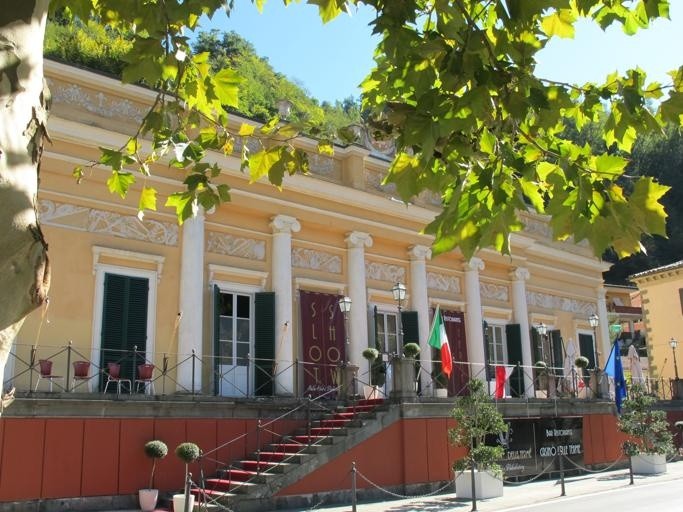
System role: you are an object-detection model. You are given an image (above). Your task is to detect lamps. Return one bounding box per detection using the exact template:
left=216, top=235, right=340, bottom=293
left=71, top=360, right=95, bottom=393
left=104, top=363, right=132, bottom=394
left=134, top=364, right=156, bottom=396
left=35, top=358, right=65, bottom=394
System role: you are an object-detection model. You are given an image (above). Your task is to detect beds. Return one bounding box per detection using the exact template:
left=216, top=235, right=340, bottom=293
left=564, top=337, right=577, bottom=391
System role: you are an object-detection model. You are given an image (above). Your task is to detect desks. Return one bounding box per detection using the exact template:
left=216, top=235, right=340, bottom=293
left=336, top=293, right=352, bottom=365
left=668, top=336, right=679, bottom=380
left=388, top=281, right=408, bottom=356
left=587, top=312, right=600, bottom=371
left=535, top=320, right=547, bottom=363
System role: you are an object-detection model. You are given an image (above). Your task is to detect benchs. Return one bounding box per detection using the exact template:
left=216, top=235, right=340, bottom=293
left=427, top=307, right=453, bottom=380
left=603, top=337, right=627, bottom=417
left=495, top=366, right=514, bottom=398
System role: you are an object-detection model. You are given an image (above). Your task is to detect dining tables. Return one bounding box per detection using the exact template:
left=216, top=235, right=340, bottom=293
left=535, top=361, right=547, bottom=398
left=138, top=440, right=168, bottom=512
left=173, top=444, right=200, bottom=512
left=574, top=357, right=589, bottom=399
left=362, top=347, right=379, bottom=400
left=402, top=343, right=420, bottom=392
left=430, top=356, right=448, bottom=398
left=445, top=378, right=513, bottom=499
left=619, top=381, right=670, bottom=474
